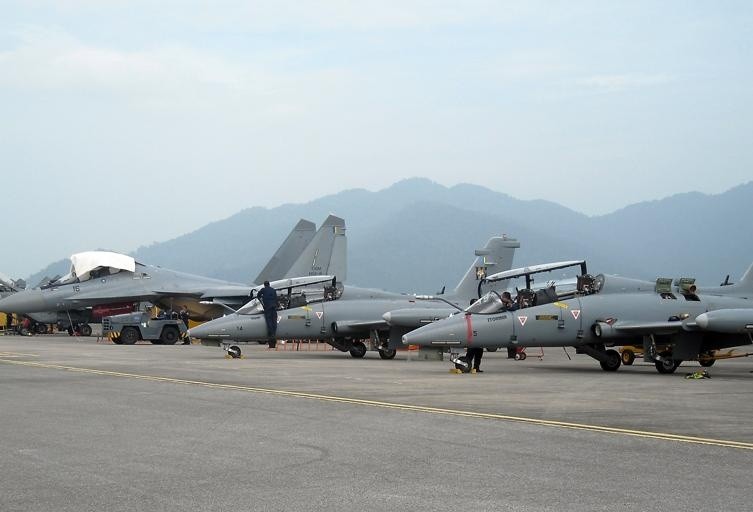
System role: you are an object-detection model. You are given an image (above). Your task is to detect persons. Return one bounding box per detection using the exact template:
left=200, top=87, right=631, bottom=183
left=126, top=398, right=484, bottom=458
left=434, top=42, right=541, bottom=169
left=256, top=281, right=278, bottom=337
left=500, top=292, right=515, bottom=311
left=465, top=297, right=484, bottom=372
left=20, top=315, right=29, bottom=335
left=179, top=304, right=190, bottom=345
left=6, top=313, right=14, bottom=326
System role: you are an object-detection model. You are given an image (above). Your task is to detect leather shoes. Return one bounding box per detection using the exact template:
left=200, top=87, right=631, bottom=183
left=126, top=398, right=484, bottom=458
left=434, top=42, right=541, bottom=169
left=473, top=367, right=483, bottom=372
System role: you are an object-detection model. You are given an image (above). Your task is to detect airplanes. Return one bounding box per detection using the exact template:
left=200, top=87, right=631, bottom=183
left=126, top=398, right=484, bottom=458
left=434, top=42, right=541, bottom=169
left=183, top=234, right=521, bottom=358
left=400, top=257, right=746, bottom=374
left=0, top=213, right=347, bottom=345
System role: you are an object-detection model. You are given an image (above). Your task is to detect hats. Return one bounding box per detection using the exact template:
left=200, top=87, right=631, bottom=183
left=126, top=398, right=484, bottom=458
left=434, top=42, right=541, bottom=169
left=501, top=292, right=514, bottom=303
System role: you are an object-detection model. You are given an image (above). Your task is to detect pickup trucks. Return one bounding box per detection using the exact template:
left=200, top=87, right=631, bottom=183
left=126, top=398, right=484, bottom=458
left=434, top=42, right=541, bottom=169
left=102, top=306, right=191, bottom=346
